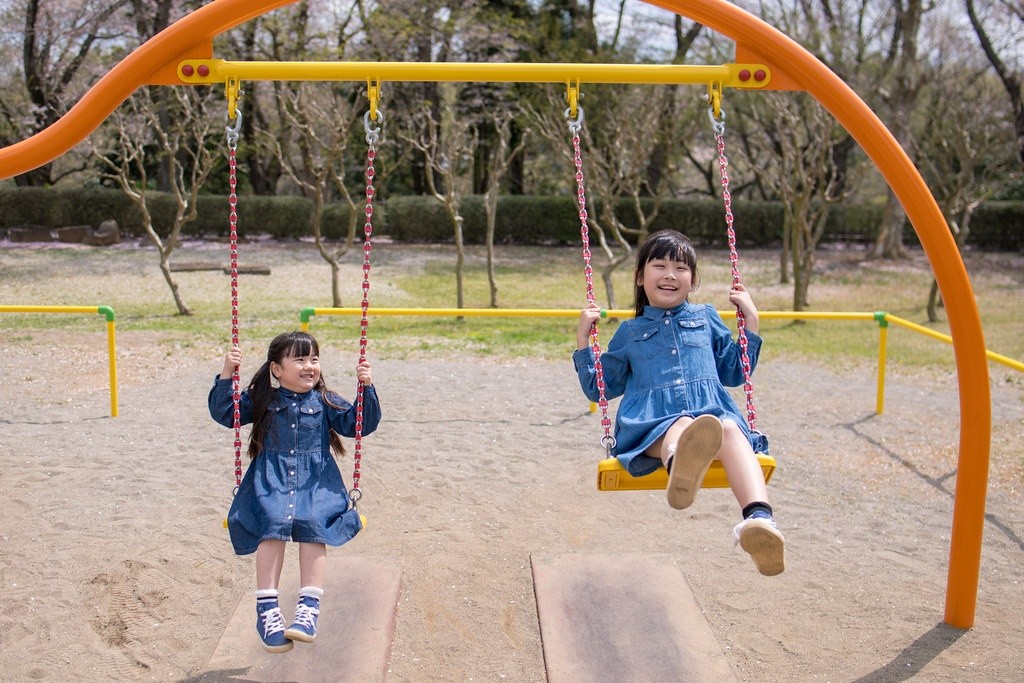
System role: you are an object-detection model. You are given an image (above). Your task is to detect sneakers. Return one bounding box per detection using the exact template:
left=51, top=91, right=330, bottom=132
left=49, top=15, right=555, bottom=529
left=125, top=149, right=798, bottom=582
left=256, top=599, right=294, bottom=652
left=284, top=595, right=320, bottom=643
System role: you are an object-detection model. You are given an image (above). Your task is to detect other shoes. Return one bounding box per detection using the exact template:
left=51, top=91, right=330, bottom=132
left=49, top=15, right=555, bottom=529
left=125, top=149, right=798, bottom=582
left=733, top=512, right=785, bottom=576
left=666, top=412, right=724, bottom=509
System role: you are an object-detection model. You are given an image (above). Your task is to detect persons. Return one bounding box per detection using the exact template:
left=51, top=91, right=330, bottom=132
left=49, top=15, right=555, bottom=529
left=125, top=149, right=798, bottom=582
left=573, top=228, right=785, bottom=576
left=207, top=331, right=381, bottom=653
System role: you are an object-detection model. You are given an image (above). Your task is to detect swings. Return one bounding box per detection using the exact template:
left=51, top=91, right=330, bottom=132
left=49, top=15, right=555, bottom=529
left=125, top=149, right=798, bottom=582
left=224, top=106, right=384, bottom=532
left=562, top=100, right=780, bottom=493
